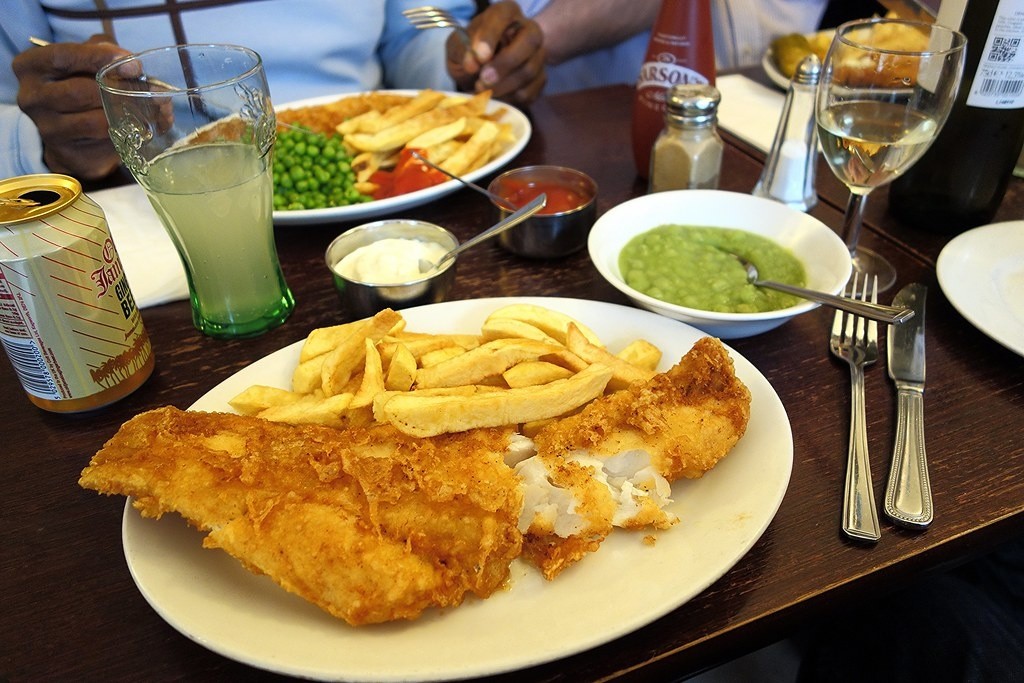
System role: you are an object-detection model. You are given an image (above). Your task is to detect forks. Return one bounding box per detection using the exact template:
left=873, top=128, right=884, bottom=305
left=402, top=5, right=468, bottom=42
left=829, top=271, right=882, bottom=543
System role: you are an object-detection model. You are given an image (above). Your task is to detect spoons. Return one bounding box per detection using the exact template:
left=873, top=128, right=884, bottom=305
left=716, top=245, right=915, bottom=326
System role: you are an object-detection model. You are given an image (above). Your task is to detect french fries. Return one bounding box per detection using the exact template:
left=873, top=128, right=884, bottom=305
left=229, top=308, right=663, bottom=439
left=335, top=89, right=517, bottom=190
left=813, top=12, right=926, bottom=76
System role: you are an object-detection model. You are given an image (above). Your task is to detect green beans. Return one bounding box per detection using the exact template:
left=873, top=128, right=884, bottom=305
left=242, top=121, right=375, bottom=211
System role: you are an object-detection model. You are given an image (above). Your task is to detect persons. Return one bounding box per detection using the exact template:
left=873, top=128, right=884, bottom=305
left=0, top=0, right=824, bottom=193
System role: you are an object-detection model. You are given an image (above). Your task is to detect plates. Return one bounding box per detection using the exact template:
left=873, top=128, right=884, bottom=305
left=935, top=220, right=1024, bottom=357
left=122, top=297, right=795, bottom=683
left=760, top=46, right=914, bottom=97
left=167, top=87, right=531, bottom=226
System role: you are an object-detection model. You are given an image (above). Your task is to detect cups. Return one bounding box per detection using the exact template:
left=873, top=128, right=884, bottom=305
left=94, top=42, right=295, bottom=340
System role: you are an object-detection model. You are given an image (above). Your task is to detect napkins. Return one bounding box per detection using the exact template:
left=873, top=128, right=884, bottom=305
left=82, top=182, right=194, bottom=309
left=709, top=74, right=828, bottom=155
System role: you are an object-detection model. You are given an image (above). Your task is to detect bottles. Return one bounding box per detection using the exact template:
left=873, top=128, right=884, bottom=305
left=630, top=0, right=716, bottom=178
left=646, top=85, right=724, bottom=192
left=753, top=53, right=820, bottom=213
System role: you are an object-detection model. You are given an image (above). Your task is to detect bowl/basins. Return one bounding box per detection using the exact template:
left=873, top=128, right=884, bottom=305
left=324, top=219, right=459, bottom=322
left=586, top=188, right=853, bottom=339
left=486, top=164, right=598, bottom=259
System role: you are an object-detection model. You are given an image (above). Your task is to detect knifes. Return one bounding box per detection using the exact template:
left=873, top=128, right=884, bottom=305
left=884, top=281, right=935, bottom=530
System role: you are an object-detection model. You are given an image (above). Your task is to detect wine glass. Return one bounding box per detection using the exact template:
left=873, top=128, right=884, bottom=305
left=815, top=18, right=968, bottom=298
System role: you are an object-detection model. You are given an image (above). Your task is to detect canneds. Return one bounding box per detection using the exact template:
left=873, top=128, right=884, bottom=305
left=0, top=173, right=155, bottom=414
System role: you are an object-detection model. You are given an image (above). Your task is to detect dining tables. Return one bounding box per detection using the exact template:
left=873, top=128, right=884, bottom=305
left=0, top=78, right=1024, bottom=683
